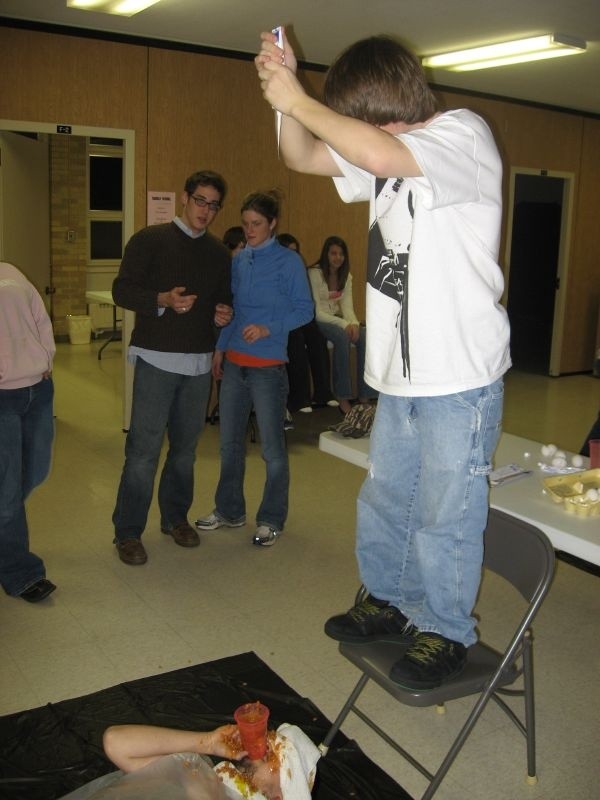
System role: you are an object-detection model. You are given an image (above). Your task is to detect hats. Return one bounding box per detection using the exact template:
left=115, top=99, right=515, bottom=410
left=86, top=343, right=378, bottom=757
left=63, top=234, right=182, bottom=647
left=327, top=404, right=378, bottom=434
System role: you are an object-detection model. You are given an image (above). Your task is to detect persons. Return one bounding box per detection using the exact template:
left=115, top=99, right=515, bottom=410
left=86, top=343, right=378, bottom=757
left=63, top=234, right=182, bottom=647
left=54, top=721, right=320, bottom=800
left=111, top=170, right=235, bottom=567
left=0, top=259, right=62, bottom=603
left=193, top=191, right=315, bottom=548
left=213, top=225, right=378, bottom=434
left=254, top=26, right=514, bottom=689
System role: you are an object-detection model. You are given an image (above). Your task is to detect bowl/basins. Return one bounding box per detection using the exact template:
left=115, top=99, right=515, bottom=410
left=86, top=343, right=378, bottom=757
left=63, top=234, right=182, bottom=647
left=541, top=469, right=600, bottom=518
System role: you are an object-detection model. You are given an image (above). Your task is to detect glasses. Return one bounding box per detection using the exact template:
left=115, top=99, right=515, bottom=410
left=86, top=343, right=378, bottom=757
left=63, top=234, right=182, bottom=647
left=190, top=194, right=221, bottom=211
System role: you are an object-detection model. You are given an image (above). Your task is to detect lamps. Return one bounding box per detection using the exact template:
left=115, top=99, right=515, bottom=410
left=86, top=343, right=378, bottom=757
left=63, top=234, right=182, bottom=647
left=66, top=0, right=161, bottom=18
left=421, top=32, right=588, bottom=73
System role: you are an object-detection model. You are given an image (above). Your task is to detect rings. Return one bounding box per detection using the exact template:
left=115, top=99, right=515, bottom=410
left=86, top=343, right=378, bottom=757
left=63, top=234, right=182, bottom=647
left=183, top=307, right=186, bottom=311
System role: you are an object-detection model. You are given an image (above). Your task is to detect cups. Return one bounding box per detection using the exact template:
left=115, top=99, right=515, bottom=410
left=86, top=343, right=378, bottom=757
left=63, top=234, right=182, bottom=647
left=590, top=440, right=600, bottom=469
left=234, top=703, right=270, bottom=760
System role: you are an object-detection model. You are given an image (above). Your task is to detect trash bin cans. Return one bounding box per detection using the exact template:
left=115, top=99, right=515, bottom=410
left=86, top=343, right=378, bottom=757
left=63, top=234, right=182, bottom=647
left=67, top=314, right=92, bottom=345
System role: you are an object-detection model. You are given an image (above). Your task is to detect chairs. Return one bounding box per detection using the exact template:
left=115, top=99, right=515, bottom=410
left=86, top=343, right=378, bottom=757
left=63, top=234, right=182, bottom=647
left=318, top=506, right=557, bottom=800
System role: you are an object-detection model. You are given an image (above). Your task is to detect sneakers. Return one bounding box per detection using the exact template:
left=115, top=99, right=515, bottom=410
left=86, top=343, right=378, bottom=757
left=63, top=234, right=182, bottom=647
left=161, top=523, right=200, bottom=547
left=323, top=585, right=403, bottom=641
left=112, top=534, right=147, bottom=564
left=389, top=633, right=469, bottom=692
left=194, top=512, right=245, bottom=529
left=253, top=525, right=279, bottom=546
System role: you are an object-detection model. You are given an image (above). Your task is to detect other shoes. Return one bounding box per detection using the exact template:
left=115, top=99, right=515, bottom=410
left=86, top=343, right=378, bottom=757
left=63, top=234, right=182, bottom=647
left=21, top=578, right=56, bottom=602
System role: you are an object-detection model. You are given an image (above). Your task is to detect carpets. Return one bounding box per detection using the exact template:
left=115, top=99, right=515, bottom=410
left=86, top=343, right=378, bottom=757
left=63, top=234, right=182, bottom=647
left=0, top=651, right=416, bottom=800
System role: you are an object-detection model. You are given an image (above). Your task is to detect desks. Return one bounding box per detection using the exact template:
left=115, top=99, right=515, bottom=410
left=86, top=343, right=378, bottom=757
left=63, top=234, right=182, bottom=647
left=209, top=342, right=356, bottom=444
left=85, top=291, right=123, bottom=360
left=319, top=430, right=600, bottom=606
